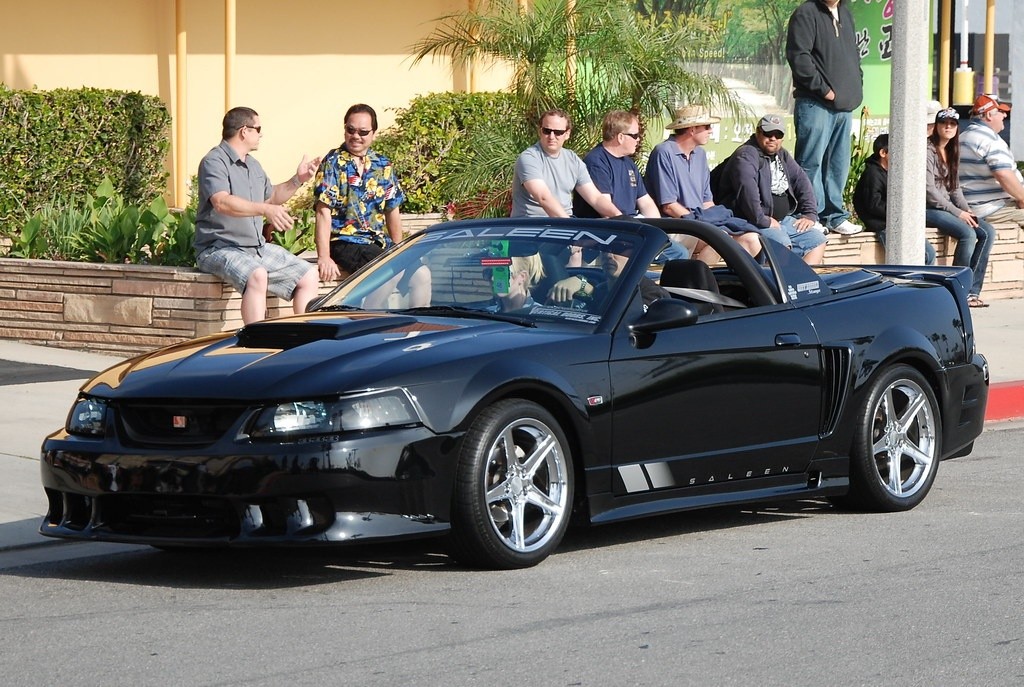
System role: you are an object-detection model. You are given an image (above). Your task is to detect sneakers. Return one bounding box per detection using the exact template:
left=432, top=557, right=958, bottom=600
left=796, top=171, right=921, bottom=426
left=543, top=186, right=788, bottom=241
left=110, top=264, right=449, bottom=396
left=829, top=220, right=863, bottom=235
left=813, top=220, right=829, bottom=235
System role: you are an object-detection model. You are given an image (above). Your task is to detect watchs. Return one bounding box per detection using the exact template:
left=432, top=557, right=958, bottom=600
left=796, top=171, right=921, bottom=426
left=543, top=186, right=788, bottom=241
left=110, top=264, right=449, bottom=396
left=576, top=274, right=588, bottom=291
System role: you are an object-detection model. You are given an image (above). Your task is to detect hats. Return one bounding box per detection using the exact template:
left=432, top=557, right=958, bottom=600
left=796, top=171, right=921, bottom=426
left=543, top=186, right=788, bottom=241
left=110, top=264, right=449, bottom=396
left=756, top=114, right=785, bottom=134
left=665, top=105, right=721, bottom=129
left=972, top=93, right=1011, bottom=115
left=935, top=107, right=960, bottom=126
left=603, top=215, right=646, bottom=245
left=926, top=100, right=942, bottom=124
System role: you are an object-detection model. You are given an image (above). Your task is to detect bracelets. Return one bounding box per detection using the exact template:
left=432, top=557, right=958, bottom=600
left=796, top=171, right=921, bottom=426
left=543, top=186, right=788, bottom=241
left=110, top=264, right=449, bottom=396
left=292, top=174, right=302, bottom=189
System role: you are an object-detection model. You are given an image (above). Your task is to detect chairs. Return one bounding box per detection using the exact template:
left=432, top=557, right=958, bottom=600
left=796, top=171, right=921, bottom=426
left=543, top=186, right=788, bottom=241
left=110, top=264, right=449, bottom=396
left=659, top=259, right=724, bottom=316
left=540, top=267, right=607, bottom=306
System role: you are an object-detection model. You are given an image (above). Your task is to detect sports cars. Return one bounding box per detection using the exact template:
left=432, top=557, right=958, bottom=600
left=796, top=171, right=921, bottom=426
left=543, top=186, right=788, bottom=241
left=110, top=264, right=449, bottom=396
left=37, top=217, right=991, bottom=569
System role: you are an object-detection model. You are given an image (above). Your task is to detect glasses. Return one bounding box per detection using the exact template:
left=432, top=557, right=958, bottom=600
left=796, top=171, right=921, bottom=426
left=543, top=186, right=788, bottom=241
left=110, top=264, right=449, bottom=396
left=345, top=123, right=372, bottom=136
left=617, top=131, right=640, bottom=140
left=540, top=126, right=568, bottom=137
left=982, top=108, right=1004, bottom=118
left=758, top=128, right=784, bottom=140
left=696, top=124, right=710, bottom=130
left=236, top=125, right=261, bottom=133
left=595, top=242, right=634, bottom=255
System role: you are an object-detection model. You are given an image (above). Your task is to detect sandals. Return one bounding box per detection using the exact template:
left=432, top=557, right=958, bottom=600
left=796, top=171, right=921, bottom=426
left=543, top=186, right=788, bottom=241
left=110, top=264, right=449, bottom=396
left=967, top=297, right=989, bottom=307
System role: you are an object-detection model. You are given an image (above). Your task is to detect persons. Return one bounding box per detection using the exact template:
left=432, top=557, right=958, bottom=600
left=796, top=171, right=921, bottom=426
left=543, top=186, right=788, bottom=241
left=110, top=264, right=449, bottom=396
left=852, top=135, right=936, bottom=265
left=958, top=95, right=1024, bottom=229
left=313, top=104, right=432, bottom=309
left=483, top=109, right=688, bottom=316
left=926, top=106, right=995, bottom=308
left=786, top=0, right=863, bottom=234
left=196, top=107, right=321, bottom=324
left=641, top=105, right=764, bottom=263
left=927, top=100, right=942, bottom=137
left=717, top=114, right=828, bottom=266
